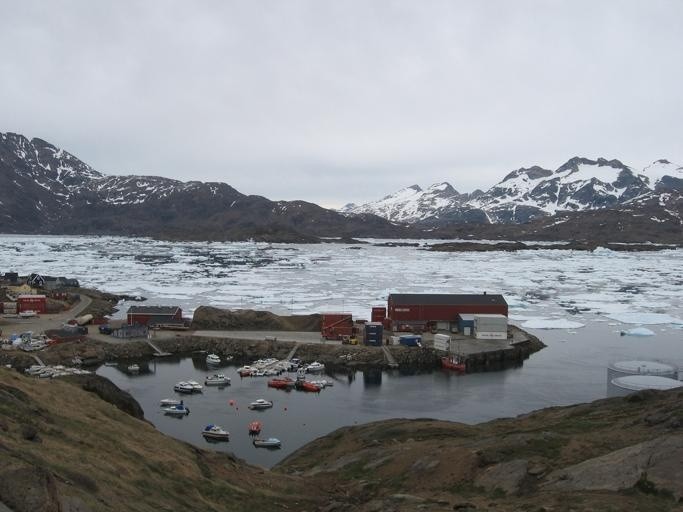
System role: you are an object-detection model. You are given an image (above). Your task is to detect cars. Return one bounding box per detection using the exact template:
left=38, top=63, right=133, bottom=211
left=431, top=326, right=437, bottom=334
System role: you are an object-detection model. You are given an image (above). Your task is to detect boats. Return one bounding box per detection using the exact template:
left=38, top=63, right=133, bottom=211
left=26, top=364, right=91, bottom=378
left=104, top=362, right=119, bottom=366
left=128, top=364, right=140, bottom=371
left=442, top=356, right=464, bottom=370
left=160, top=352, right=334, bottom=449
